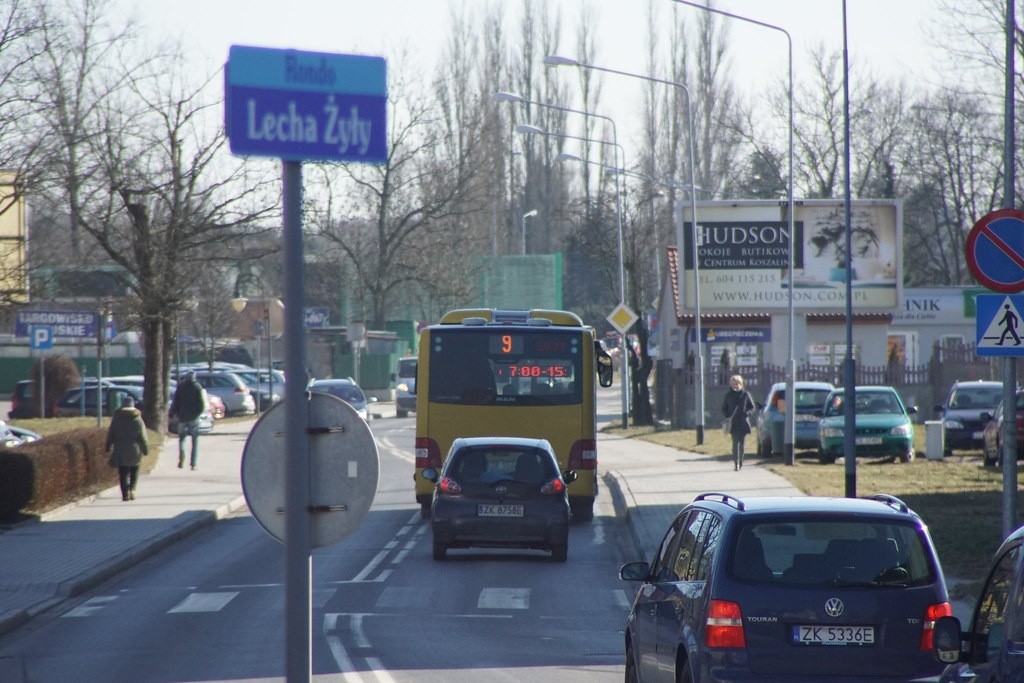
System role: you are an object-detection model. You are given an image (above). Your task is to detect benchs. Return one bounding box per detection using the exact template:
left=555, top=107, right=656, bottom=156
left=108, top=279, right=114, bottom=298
left=745, top=563, right=907, bottom=589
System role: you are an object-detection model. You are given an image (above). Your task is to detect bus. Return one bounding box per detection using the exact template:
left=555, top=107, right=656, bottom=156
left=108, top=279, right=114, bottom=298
left=415, top=308, right=613, bottom=524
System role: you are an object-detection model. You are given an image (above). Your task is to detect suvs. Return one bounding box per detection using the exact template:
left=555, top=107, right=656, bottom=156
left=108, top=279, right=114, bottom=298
left=421, top=437, right=578, bottom=562
left=935, top=379, right=1019, bottom=454
left=9, top=380, right=116, bottom=419
left=172, top=361, right=285, bottom=419
left=619, top=492, right=959, bottom=683
left=309, top=376, right=377, bottom=426
left=935, top=527, right=1024, bottom=683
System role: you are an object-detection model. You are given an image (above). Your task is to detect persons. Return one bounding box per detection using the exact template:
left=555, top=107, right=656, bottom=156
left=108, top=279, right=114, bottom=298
left=169, top=371, right=205, bottom=470
left=105, top=397, right=149, bottom=502
left=722, top=375, right=756, bottom=471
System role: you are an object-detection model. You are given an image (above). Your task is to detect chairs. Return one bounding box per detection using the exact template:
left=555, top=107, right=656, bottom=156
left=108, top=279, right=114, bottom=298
left=955, top=394, right=971, bottom=408
left=823, top=538, right=856, bottom=564
left=461, top=454, right=486, bottom=477
left=517, top=454, right=539, bottom=474
left=992, top=393, right=1002, bottom=405
left=740, top=538, right=763, bottom=574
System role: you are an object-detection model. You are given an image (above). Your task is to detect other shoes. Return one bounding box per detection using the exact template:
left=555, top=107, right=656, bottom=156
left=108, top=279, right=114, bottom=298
left=179, top=464, right=184, bottom=468
left=191, top=466, right=195, bottom=469
left=130, top=489, right=136, bottom=500
left=123, top=496, right=129, bottom=501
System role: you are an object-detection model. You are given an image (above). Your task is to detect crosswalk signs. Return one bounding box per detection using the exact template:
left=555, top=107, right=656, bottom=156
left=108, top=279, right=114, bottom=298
left=975, top=293, right=1024, bottom=358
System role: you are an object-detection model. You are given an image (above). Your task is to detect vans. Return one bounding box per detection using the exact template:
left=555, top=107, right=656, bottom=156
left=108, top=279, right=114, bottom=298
left=394, top=357, right=417, bottom=417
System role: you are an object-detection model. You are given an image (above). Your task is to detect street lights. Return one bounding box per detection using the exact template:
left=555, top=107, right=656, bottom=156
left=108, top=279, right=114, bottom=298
left=517, top=124, right=629, bottom=231
left=543, top=53, right=706, bottom=444
left=497, top=90, right=628, bottom=431
left=522, top=209, right=538, bottom=256
left=563, top=153, right=660, bottom=289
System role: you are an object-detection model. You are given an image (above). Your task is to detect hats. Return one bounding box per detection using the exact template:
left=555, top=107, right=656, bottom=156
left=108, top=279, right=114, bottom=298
left=121, top=397, right=134, bottom=407
left=185, top=370, right=197, bottom=380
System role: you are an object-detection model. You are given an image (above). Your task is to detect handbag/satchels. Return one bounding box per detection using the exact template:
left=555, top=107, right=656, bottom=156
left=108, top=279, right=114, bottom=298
left=720, top=418, right=732, bottom=434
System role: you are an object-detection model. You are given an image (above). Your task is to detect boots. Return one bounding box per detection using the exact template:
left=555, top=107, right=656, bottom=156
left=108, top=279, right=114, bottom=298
left=734, top=461, right=739, bottom=471
left=740, top=459, right=743, bottom=469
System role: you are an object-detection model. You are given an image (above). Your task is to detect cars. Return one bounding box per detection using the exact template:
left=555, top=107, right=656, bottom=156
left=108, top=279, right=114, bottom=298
left=107, top=377, right=224, bottom=420
left=980, top=390, right=1024, bottom=466
left=813, top=386, right=918, bottom=464
left=755, top=382, right=840, bottom=457
left=0, top=420, right=41, bottom=450
left=54, top=386, right=215, bottom=435
